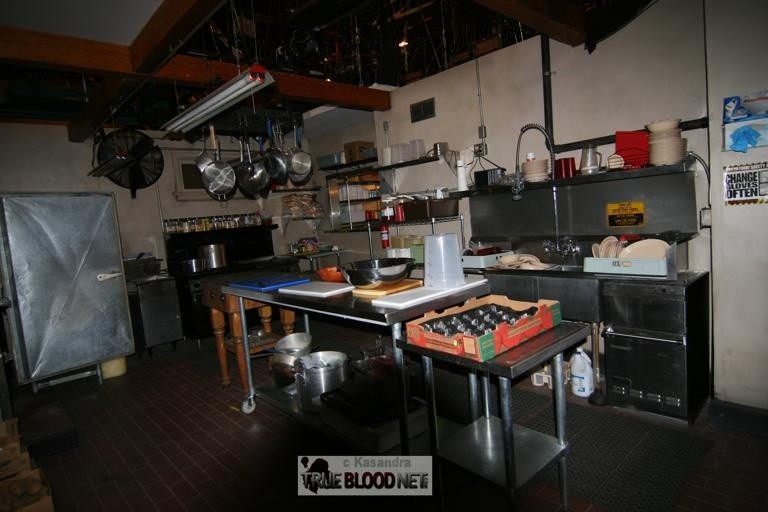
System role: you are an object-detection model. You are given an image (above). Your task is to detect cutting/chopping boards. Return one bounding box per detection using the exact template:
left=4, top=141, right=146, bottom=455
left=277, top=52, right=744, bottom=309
left=351, top=279, right=422, bottom=296
left=227, top=273, right=310, bottom=292
left=277, top=281, right=353, bottom=299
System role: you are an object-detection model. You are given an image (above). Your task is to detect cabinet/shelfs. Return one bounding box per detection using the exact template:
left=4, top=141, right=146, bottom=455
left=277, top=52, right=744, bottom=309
left=204, top=281, right=296, bottom=399
left=318, top=157, right=461, bottom=234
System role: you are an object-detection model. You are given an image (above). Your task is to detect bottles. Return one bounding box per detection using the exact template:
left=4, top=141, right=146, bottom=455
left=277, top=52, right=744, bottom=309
left=162, top=212, right=261, bottom=234
left=364, top=209, right=379, bottom=221
left=456, top=160, right=468, bottom=192
left=578, top=143, right=602, bottom=176
left=379, top=121, right=391, bottom=166
left=288, top=241, right=305, bottom=254
left=380, top=198, right=395, bottom=223
left=395, top=203, right=405, bottom=222
left=525, top=152, right=534, bottom=161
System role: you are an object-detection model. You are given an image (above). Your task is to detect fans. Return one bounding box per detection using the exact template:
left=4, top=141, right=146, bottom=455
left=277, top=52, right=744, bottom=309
left=98, top=129, right=164, bottom=198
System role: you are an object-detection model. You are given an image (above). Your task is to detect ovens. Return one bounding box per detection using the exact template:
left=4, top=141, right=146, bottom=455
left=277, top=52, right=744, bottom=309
left=600, top=280, right=711, bottom=427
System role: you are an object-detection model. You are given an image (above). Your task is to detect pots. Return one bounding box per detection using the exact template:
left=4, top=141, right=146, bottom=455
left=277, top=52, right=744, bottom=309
left=292, top=349, right=350, bottom=415
left=123, top=251, right=164, bottom=282
left=195, top=118, right=312, bottom=201
left=180, top=258, right=206, bottom=273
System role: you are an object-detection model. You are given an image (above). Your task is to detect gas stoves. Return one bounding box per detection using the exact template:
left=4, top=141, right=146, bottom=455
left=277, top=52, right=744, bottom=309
left=172, top=256, right=309, bottom=292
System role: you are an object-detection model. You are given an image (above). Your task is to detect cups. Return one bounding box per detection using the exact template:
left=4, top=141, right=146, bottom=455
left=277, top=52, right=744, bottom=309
left=337, top=184, right=369, bottom=202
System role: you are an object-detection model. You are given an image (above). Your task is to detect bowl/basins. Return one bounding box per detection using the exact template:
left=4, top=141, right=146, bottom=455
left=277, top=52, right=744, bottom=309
left=315, top=266, right=346, bottom=284
left=641, top=119, right=681, bottom=132
left=317, top=244, right=332, bottom=251
left=274, top=332, right=312, bottom=356
left=335, top=257, right=415, bottom=289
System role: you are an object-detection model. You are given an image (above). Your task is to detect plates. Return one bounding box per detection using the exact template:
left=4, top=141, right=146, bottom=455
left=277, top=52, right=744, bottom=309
left=646, top=129, right=688, bottom=167
left=590, top=235, right=669, bottom=261
left=384, top=248, right=410, bottom=259
left=338, top=203, right=365, bottom=224
left=520, top=159, right=549, bottom=184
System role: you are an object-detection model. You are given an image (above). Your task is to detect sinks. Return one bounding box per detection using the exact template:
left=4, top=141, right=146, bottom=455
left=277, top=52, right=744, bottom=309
left=535, top=264, right=603, bottom=324
left=484, top=265, right=559, bottom=303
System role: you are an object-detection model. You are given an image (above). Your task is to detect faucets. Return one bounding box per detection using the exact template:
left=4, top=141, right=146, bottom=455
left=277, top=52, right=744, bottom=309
left=542, top=238, right=581, bottom=255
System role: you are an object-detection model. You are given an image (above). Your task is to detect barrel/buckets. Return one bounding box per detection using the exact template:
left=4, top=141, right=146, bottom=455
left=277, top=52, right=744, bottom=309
left=100, top=356, right=128, bottom=380
left=569, top=347, right=595, bottom=398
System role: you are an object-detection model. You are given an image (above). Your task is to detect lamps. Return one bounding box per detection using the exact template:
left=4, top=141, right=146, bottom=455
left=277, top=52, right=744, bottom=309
left=159, top=0, right=275, bottom=133
left=89, top=151, right=131, bottom=177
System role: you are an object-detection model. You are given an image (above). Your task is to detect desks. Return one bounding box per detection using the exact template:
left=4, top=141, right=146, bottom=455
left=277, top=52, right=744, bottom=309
left=396, top=318, right=595, bottom=511
left=281, top=247, right=347, bottom=271
